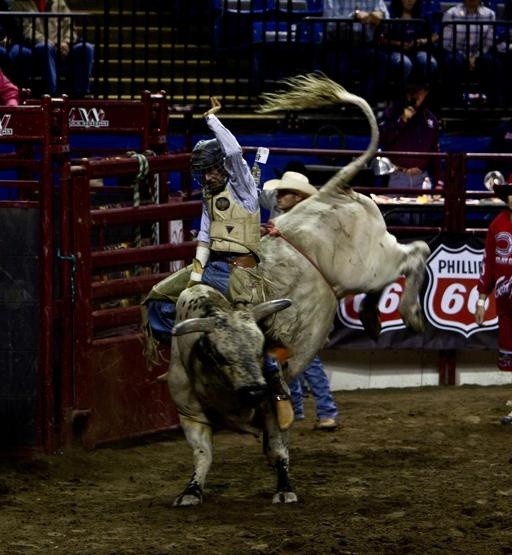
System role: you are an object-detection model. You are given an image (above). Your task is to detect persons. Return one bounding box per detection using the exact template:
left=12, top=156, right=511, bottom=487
left=474, top=173, right=512, bottom=425
left=1, top=0, right=36, bottom=82
left=144, top=97, right=295, bottom=430
left=440, top=0, right=499, bottom=92
left=1, top=69, right=24, bottom=107
left=323, top=0, right=392, bottom=83
left=374, top=0, right=443, bottom=91
left=10, top=0, right=97, bottom=97
left=261, top=169, right=338, bottom=431
left=247, top=156, right=310, bottom=219
left=381, top=78, right=441, bottom=222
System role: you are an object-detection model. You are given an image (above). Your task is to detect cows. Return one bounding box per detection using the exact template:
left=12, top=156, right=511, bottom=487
left=165, top=70, right=431, bottom=508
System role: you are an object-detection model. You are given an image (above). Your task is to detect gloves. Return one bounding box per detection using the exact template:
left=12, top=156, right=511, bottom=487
left=187, top=258, right=208, bottom=287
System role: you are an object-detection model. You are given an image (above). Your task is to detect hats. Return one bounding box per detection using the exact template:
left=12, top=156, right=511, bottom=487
left=262, top=170, right=319, bottom=196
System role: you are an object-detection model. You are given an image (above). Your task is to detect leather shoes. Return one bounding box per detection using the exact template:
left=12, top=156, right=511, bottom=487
left=275, top=393, right=295, bottom=432
left=314, top=414, right=339, bottom=430
left=157, top=372, right=170, bottom=381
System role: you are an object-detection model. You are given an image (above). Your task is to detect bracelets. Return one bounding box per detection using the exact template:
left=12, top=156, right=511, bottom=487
left=476, top=299, right=484, bottom=309
left=189, top=271, right=201, bottom=284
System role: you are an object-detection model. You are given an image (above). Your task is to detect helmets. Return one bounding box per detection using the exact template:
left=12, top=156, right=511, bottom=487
left=188, top=138, right=227, bottom=192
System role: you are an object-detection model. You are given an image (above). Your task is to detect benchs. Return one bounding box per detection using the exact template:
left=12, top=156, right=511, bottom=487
left=211, top=2, right=512, bottom=46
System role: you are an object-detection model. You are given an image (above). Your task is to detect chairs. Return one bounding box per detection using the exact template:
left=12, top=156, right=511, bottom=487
left=0, top=128, right=511, bottom=229
